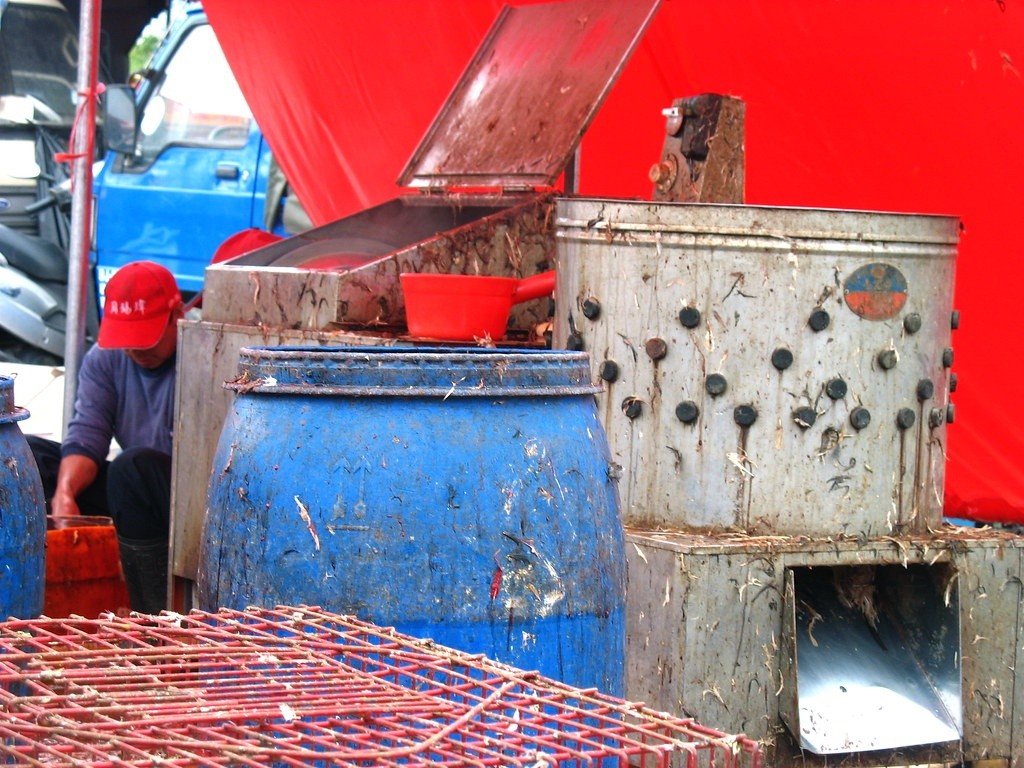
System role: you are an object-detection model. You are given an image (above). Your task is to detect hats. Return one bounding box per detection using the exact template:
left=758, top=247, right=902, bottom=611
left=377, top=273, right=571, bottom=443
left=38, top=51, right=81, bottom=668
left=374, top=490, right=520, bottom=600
left=97, top=262, right=180, bottom=350
left=183, top=226, right=286, bottom=312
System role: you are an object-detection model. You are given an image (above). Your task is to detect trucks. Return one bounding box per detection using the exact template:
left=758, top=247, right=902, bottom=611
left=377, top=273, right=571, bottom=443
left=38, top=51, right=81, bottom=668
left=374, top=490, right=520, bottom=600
left=0, top=1, right=114, bottom=235
left=81, top=5, right=311, bottom=329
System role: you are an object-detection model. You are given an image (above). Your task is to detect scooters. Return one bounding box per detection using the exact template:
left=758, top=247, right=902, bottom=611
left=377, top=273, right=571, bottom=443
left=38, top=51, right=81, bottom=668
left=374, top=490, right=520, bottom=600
left=0, top=161, right=105, bottom=367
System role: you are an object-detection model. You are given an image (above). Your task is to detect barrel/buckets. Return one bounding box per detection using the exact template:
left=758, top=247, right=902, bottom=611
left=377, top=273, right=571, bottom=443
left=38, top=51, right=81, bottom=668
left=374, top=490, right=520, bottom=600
left=46, top=513, right=129, bottom=625
left=194, top=346, right=630, bottom=768
left=0, top=375, right=47, bottom=764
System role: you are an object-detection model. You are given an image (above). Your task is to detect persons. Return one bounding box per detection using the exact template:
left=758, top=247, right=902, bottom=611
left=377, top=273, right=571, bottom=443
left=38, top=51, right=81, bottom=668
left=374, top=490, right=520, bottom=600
left=51, top=261, right=183, bottom=616
